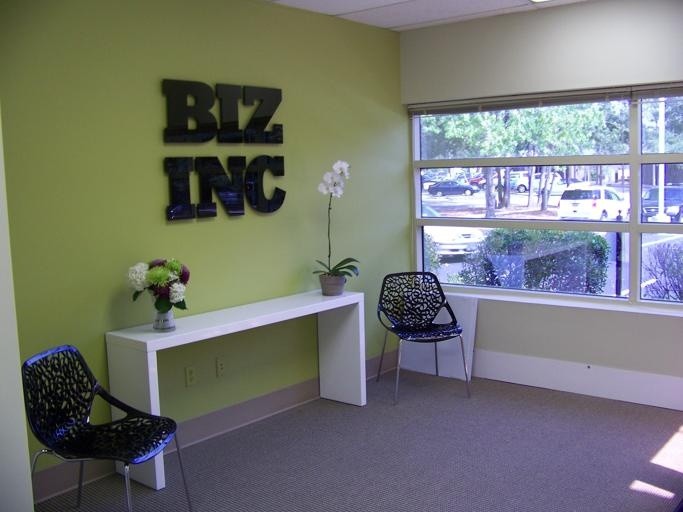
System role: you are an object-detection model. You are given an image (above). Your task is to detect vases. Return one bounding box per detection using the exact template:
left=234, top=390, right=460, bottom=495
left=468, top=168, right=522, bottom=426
left=311, top=159, right=360, bottom=275
left=148, top=297, right=176, bottom=333
left=318, top=275, right=344, bottom=297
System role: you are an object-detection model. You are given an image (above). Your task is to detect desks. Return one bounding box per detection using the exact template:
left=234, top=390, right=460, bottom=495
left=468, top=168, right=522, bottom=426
left=106, top=286, right=366, bottom=490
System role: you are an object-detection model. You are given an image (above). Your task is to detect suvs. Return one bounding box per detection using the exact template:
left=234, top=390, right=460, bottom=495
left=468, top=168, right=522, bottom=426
left=629, top=183, right=681, bottom=224
left=554, top=181, right=629, bottom=228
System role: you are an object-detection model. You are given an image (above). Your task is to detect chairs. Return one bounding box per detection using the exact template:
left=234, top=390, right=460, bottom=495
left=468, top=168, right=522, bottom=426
left=20, top=343, right=193, bottom=512
left=374, top=269, right=473, bottom=406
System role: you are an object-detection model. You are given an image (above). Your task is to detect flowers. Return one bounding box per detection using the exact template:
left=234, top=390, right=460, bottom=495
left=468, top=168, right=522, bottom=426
left=127, top=257, right=192, bottom=311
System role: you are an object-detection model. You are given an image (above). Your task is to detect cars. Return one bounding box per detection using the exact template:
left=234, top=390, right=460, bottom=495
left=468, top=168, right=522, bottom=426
left=420, top=166, right=563, bottom=204
left=421, top=202, right=485, bottom=264
